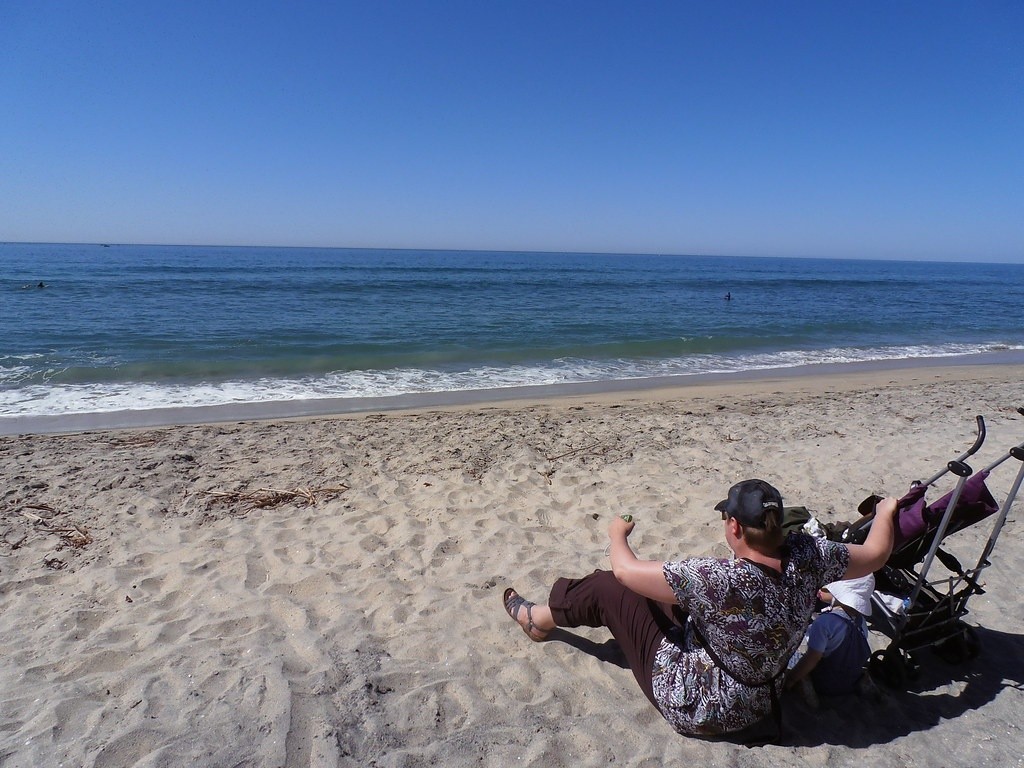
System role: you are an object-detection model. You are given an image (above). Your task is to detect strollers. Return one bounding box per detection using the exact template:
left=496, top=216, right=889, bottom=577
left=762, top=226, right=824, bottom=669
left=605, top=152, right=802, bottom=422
left=809, top=407, right=1024, bottom=693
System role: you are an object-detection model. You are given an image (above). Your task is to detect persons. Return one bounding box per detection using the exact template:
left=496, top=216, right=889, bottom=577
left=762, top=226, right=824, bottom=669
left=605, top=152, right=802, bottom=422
left=785, top=572, right=876, bottom=698
left=503, top=479, right=897, bottom=735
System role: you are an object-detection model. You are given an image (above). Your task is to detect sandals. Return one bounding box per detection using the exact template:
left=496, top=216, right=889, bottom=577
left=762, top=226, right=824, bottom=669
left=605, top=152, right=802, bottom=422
left=502, top=588, right=551, bottom=642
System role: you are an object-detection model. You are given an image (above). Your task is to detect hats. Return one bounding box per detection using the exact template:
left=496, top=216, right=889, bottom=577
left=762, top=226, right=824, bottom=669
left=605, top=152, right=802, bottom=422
left=714, top=479, right=784, bottom=527
left=822, top=572, right=876, bottom=615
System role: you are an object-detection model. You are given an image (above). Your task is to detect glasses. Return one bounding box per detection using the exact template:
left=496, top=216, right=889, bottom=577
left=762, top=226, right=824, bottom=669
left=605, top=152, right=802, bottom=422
left=721, top=511, right=731, bottom=520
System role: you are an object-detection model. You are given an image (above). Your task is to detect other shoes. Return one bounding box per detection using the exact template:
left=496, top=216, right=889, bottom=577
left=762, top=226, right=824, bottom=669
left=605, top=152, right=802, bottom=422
left=795, top=671, right=818, bottom=709
left=854, top=670, right=875, bottom=695
